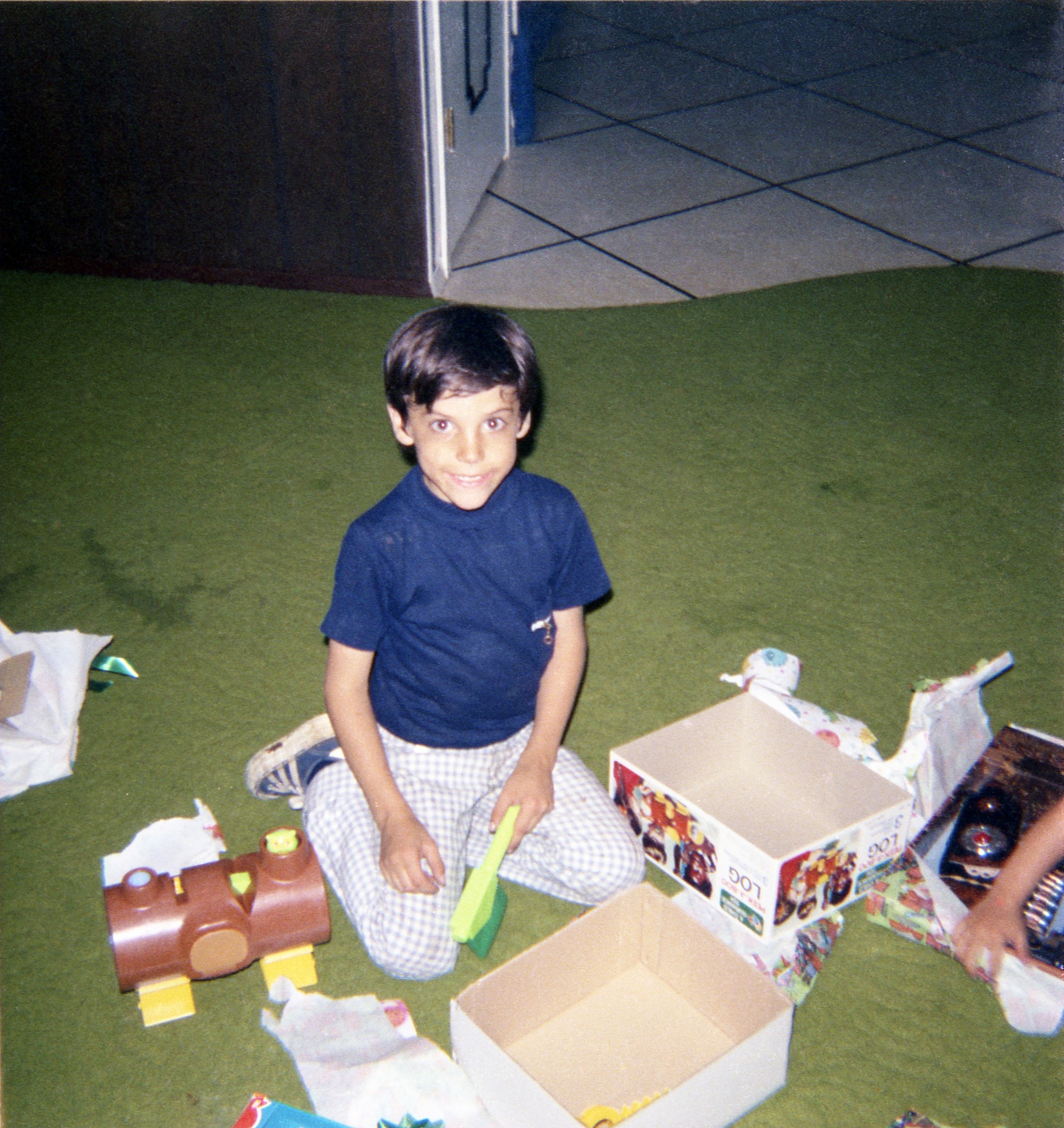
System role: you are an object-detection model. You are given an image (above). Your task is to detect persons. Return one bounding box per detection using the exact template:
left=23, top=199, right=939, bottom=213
left=240, top=298, right=640, bottom=980
left=950, top=795, right=1064, bottom=977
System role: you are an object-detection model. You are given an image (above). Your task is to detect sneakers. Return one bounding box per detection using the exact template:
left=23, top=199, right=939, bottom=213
left=244, top=711, right=348, bottom=801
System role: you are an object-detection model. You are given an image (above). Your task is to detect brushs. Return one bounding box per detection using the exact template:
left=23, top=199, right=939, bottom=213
left=451, top=805, right=523, bottom=959
